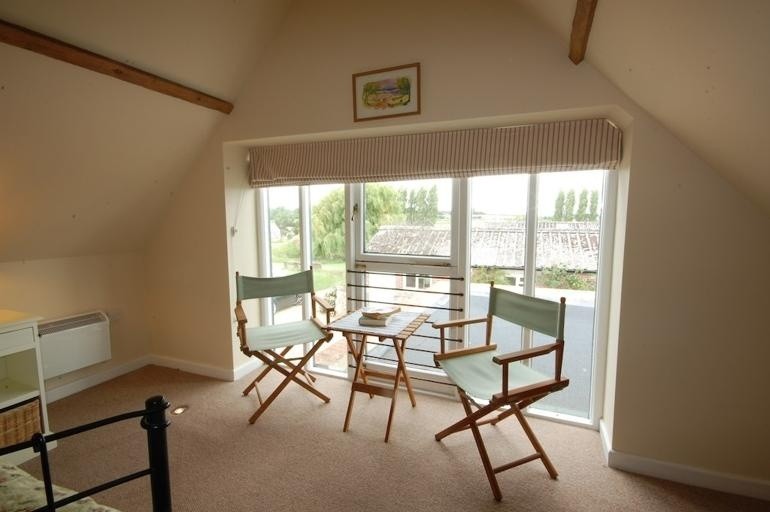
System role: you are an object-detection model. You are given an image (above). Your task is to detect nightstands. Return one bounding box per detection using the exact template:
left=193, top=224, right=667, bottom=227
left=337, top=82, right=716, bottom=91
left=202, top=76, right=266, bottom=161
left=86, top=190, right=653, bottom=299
left=0, top=321, right=57, bottom=467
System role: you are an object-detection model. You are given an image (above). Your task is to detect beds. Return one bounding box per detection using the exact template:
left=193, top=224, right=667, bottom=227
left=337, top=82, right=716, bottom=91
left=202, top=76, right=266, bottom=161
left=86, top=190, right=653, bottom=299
left=0, top=394, right=175, bottom=512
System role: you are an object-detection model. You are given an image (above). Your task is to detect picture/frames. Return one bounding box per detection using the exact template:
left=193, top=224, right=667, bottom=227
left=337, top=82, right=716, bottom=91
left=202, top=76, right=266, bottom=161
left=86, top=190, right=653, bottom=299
left=351, top=62, right=421, bottom=122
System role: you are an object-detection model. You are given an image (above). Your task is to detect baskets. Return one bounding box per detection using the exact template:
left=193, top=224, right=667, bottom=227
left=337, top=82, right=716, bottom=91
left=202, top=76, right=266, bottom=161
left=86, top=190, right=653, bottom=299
left=0, top=399, right=42, bottom=448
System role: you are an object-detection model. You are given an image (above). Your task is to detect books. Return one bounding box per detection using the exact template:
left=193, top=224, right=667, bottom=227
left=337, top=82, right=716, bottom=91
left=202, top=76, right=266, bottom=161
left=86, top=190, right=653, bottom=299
left=359, top=315, right=393, bottom=327
left=362, top=304, right=402, bottom=319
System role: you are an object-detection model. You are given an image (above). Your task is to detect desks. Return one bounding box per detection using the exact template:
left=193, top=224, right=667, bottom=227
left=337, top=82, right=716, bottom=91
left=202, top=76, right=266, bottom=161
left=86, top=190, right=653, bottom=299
left=319, top=307, right=430, bottom=443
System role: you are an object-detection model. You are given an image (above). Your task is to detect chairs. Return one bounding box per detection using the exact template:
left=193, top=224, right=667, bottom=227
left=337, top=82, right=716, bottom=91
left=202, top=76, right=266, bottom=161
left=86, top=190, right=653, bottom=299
left=431, top=280, right=570, bottom=501
left=231, top=264, right=337, bottom=425
